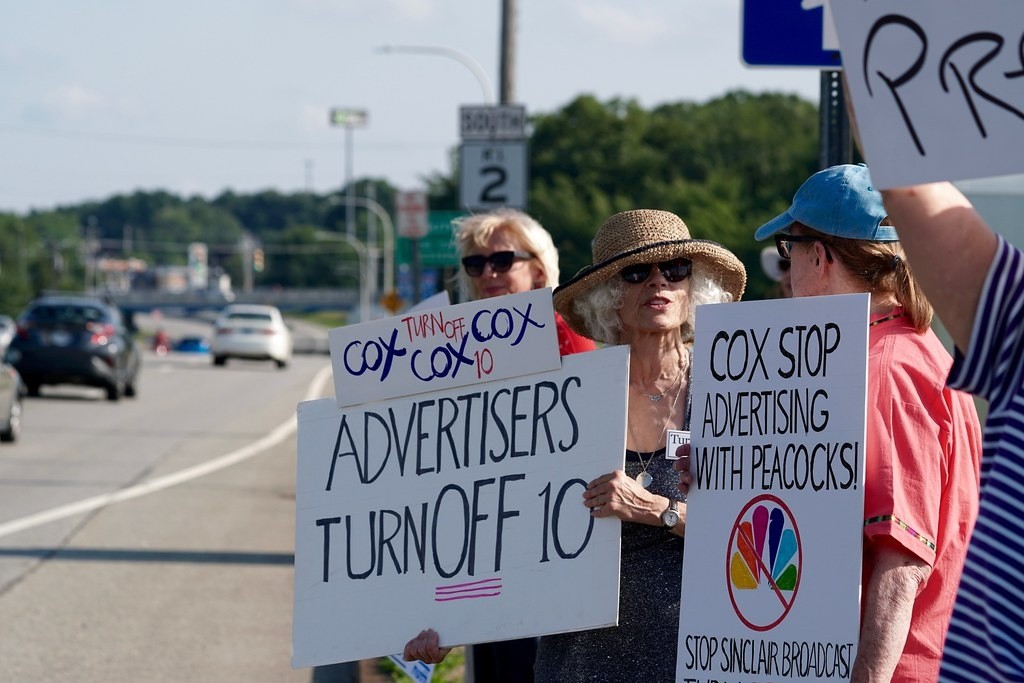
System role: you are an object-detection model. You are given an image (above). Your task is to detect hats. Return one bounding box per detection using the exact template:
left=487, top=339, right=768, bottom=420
left=755, top=163, right=899, bottom=241
left=553, top=209, right=747, bottom=344
left=761, top=247, right=789, bottom=283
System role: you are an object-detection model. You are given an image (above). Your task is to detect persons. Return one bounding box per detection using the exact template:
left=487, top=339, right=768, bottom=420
left=404, top=67, right=1024, bottom=683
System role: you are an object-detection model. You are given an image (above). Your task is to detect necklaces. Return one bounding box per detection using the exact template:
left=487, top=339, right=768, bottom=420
left=639, top=365, right=684, bottom=402
left=628, top=378, right=683, bottom=488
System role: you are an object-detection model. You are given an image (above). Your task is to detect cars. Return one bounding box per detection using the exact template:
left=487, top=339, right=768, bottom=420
left=0, top=311, right=27, bottom=443
left=10, top=297, right=142, bottom=401
left=212, top=304, right=293, bottom=368
left=176, top=336, right=213, bottom=356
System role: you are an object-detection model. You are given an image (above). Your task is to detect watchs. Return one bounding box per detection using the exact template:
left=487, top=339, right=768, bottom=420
left=662, top=500, right=679, bottom=531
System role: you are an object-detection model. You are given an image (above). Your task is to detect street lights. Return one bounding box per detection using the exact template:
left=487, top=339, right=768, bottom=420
left=378, top=44, right=494, bottom=105
left=328, top=109, right=372, bottom=238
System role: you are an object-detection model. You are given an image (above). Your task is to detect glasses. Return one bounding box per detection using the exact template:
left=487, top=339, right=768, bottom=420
left=779, top=260, right=791, bottom=272
left=620, top=257, right=692, bottom=284
left=462, top=250, right=535, bottom=278
left=774, top=233, right=833, bottom=265
